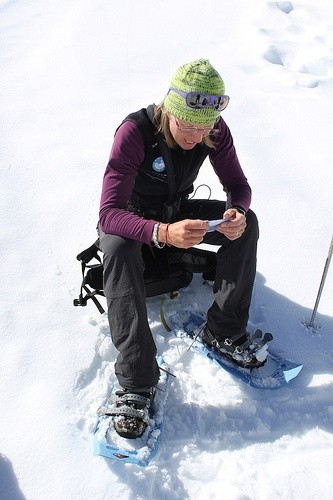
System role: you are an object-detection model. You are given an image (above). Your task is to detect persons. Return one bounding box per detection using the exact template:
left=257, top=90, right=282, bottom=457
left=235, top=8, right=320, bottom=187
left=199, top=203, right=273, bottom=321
left=94, top=58, right=303, bottom=467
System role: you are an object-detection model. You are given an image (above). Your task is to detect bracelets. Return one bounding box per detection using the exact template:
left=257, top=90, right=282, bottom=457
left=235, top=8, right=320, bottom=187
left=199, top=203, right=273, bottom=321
left=165, top=223, right=172, bottom=247
left=152, top=222, right=165, bottom=249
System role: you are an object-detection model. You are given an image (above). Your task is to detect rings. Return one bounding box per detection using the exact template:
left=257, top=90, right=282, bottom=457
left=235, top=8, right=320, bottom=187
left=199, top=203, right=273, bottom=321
left=235, top=232, right=239, bottom=236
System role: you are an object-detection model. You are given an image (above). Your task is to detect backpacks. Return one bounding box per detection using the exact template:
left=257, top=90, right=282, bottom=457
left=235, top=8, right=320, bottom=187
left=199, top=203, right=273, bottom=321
left=73, top=241, right=217, bottom=315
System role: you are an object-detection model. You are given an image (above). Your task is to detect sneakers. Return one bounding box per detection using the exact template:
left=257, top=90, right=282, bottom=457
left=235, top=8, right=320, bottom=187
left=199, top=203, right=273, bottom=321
left=202, top=325, right=273, bottom=368
left=104, top=387, right=154, bottom=439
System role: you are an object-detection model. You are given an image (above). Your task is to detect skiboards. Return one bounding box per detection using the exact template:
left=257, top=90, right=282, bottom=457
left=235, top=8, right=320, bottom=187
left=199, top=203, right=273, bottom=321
left=91, top=307, right=303, bottom=468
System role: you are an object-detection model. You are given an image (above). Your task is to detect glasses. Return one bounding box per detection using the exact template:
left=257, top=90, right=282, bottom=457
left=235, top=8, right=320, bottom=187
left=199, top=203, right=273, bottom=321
left=173, top=118, right=214, bottom=134
left=166, top=87, right=230, bottom=111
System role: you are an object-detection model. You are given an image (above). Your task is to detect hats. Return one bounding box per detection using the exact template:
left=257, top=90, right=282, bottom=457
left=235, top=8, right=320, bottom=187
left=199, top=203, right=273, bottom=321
left=162, top=58, right=225, bottom=125
